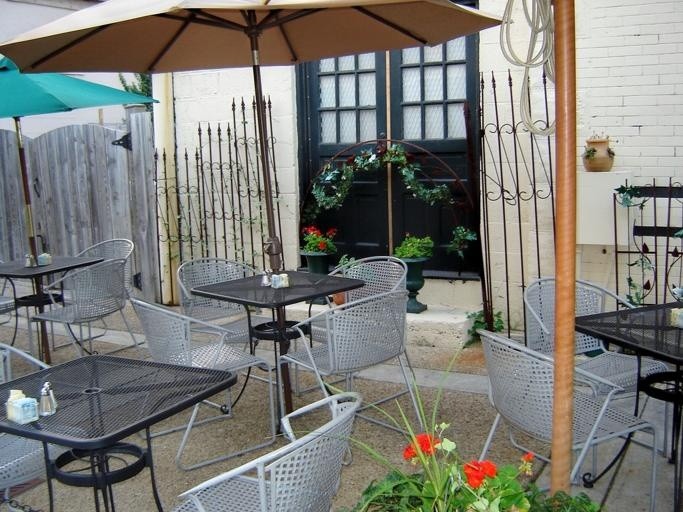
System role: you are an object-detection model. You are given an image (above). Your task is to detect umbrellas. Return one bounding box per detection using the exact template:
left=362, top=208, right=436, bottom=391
left=0, top=53, right=161, bottom=365
left=0, top=0, right=518, bottom=418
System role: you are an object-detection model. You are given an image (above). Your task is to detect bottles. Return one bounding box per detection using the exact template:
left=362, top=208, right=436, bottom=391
left=37, top=382, right=58, bottom=416
left=262, top=269, right=271, bottom=287
left=24, top=253, right=37, bottom=268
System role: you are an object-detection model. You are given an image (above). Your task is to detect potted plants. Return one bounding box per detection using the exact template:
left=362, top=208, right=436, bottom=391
left=390, top=234, right=434, bottom=314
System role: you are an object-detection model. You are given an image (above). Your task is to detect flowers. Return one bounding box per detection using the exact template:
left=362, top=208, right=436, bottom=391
left=300, top=226, right=337, bottom=253
left=266, top=329, right=608, bottom=512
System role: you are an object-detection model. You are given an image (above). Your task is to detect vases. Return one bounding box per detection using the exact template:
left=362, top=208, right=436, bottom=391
left=580, top=138, right=615, bottom=172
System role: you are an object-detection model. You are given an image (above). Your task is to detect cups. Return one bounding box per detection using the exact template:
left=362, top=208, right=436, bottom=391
left=272, top=273, right=289, bottom=289
left=37, top=254, right=51, bottom=265
